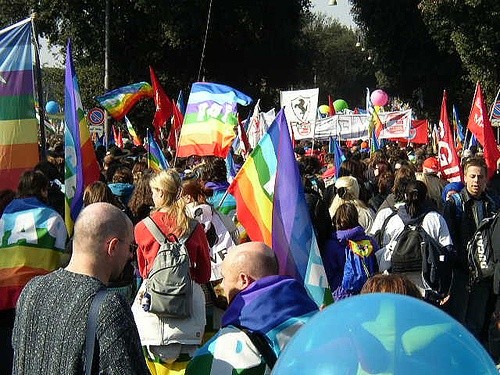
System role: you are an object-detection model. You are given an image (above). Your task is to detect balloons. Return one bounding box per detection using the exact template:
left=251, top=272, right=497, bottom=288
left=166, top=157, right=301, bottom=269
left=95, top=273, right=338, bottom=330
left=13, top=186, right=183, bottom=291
left=332, top=99, right=348, bottom=112
left=371, top=88, right=388, bottom=107
left=47, top=102, right=60, bottom=114
left=271, top=293, right=499, bottom=375
left=319, top=104, right=329, bottom=119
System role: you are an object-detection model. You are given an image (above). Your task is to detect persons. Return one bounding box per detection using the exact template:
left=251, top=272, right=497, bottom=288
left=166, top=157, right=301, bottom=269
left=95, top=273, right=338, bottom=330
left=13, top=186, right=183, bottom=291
left=182, top=242, right=322, bottom=375
left=9, top=202, right=153, bottom=374
left=441, top=161, right=500, bottom=342
left=0, top=133, right=500, bottom=375
left=379, top=179, right=454, bottom=308
left=128, top=168, right=215, bottom=366
left=0, top=169, right=72, bottom=375
left=325, top=201, right=378, bottom=304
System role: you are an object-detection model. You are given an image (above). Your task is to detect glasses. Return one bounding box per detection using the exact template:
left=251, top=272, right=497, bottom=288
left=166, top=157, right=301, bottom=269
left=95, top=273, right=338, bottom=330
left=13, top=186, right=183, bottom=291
left=107, top=236, right=138, bottom=253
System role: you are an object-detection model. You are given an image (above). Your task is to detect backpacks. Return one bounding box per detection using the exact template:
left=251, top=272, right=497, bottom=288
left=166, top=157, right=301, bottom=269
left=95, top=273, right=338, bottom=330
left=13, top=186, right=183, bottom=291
left=390, top=206, right=426, bottom=288
left=340, top=239, right=379, bottom=293
left=141, top=217, right=199, bottom=322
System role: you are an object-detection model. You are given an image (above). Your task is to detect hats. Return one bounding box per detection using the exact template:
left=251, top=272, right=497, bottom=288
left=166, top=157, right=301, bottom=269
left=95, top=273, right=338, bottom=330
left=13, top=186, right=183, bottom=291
left=424, top=157, right=439, bottom=169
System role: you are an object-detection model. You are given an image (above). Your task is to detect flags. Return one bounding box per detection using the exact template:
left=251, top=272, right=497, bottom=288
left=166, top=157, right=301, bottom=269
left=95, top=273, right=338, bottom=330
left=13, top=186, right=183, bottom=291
left=92, top=64, right=431, bottom=158
left=226, top=104, right=334, bottom=312
left=65, top=39, right=104, bottom=239
left=435, top=80, right=500, bottom=183
left=0, top=11, right=46, bottom=194
